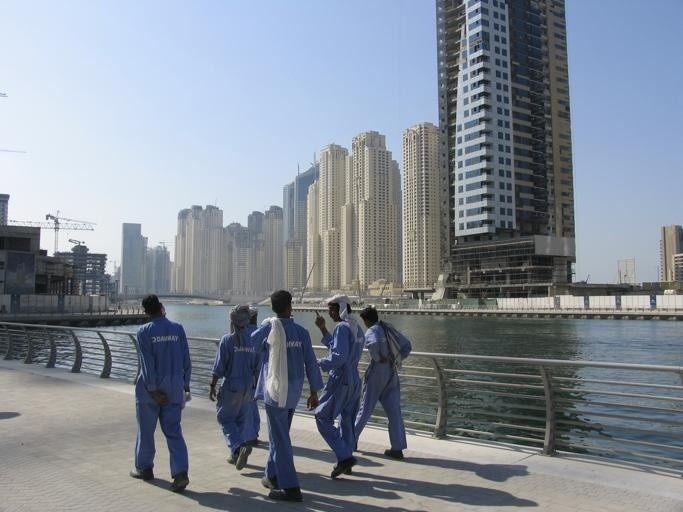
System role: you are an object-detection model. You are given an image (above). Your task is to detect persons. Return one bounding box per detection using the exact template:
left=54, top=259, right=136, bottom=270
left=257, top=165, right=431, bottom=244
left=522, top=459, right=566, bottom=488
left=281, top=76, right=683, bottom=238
left=246, top=305, right=261, bottom=448
left=207, top=303, right=260, bottom=472
left=248, top=289, right=323, bottom=501
left=126, top=293, right=191, bottom=493
left=309, top=295, right=365, bottom=479
left=352, top=304, right=411, bottom=460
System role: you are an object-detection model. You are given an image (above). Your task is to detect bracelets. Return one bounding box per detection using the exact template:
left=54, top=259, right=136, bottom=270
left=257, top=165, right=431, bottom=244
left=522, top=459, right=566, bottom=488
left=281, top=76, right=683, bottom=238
left=210, top=384, right=214, bottom=387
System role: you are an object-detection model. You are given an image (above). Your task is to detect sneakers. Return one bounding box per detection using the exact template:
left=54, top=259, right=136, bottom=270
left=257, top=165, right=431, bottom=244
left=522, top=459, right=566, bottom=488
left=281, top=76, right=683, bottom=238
left=170, top=474, right=189, bottom=490
left=261, top=478, right=277, bottom=488
left=236, top=446, right=252, bottom=469
left=130, top=468, right=154, bottom=479
left=331, top=458, right=357, bottom=478
left=269, top=491, right=304, bottom=501
left=226, top=455, right=238, bottom=465
left=384, top=449, right=403, bottom=459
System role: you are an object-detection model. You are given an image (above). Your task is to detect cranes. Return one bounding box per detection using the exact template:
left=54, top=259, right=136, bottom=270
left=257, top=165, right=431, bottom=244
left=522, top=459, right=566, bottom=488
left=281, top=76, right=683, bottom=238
left=8, top=210, right=97, bottom=253
